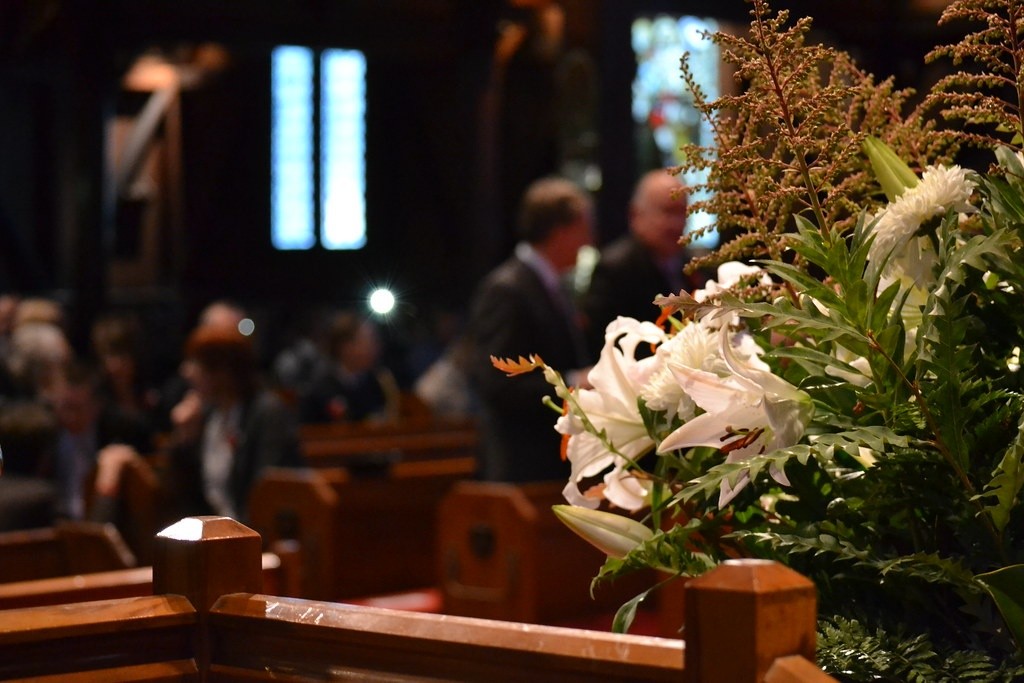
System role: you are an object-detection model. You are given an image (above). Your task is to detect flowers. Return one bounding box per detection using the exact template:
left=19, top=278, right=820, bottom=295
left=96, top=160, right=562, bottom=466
left=490, top=1, right=1024, bottom=683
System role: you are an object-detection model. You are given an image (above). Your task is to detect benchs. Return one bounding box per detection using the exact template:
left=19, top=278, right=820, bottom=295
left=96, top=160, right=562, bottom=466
left=1, top=397, right=841, bottom=681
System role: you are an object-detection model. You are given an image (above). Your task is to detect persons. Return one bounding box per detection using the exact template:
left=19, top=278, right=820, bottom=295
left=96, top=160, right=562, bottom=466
left=0, top=168, right=696, bottom=522
